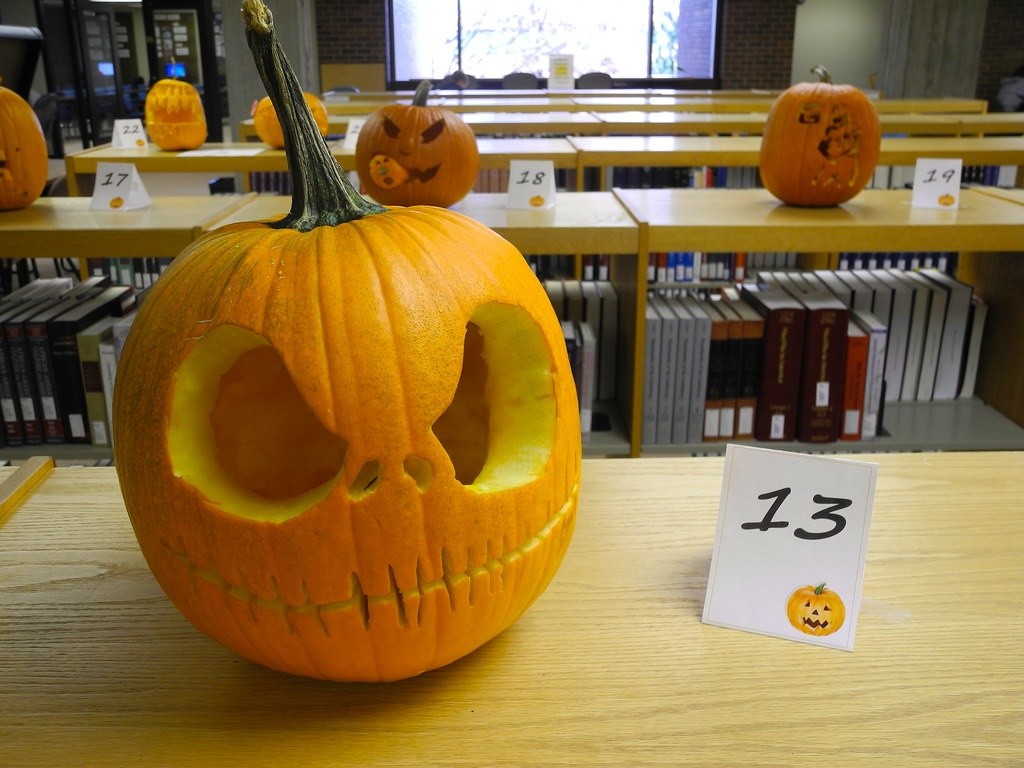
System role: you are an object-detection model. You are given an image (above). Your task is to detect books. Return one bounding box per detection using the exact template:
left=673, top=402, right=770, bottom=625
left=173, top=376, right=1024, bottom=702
left=613, top=165, right=999, bottom=459
left=0, top=134, right=291, bottom=465
left=476, top=133, right=616, bottom=431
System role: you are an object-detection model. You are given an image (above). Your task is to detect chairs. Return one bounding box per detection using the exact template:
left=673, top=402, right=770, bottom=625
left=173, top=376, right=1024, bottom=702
left=576, top=72, right=614, bottom=90
left=503, top=72, right=540, bottom=90
left=443, top=73, right=479, bottom=90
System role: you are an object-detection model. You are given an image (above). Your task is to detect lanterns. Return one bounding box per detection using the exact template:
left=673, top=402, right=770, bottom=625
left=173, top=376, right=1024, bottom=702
left=112, top=208, right=582, bottom=683
left=760, top=79, right=880, bottom=205
left=355, top=105, right=479, bottom=207
left=254, top=92, right=329, bottom=148
left=145, top=80, right=206, bottom=149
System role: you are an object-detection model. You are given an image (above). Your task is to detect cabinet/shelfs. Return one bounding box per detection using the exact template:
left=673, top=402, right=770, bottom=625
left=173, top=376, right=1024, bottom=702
left=0, top=0, right=1024, bottom=768
